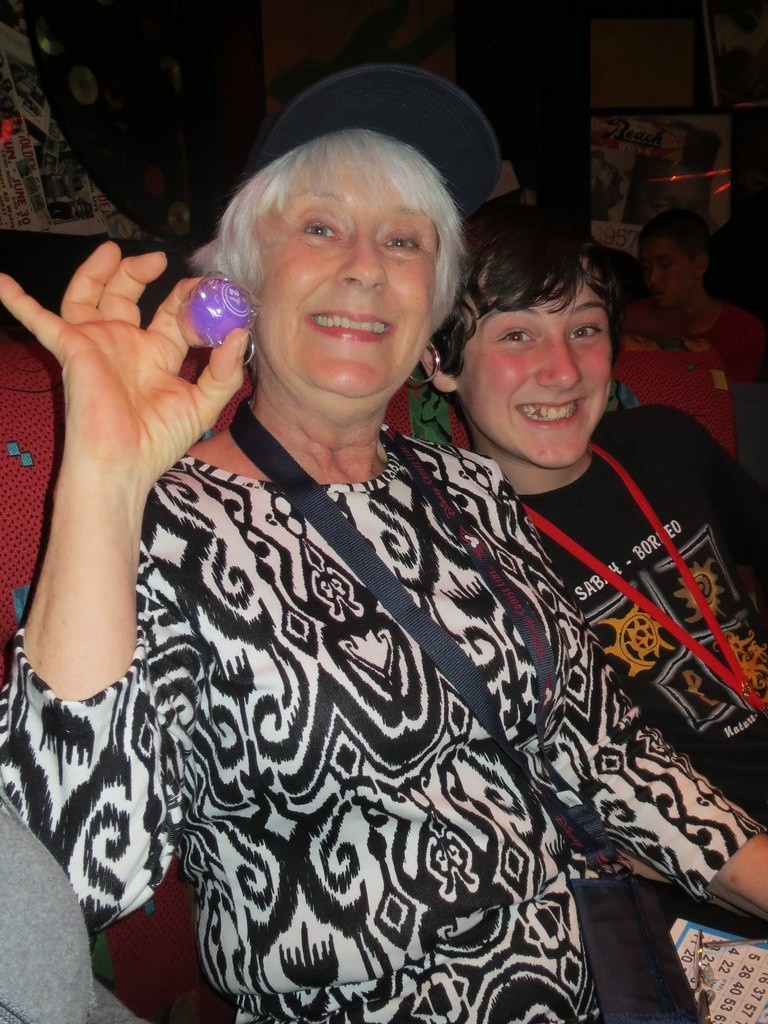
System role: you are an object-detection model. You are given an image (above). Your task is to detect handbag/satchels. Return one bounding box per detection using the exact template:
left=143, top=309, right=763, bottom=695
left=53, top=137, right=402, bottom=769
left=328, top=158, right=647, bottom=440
left=569, top=870, right=710, bottom=1024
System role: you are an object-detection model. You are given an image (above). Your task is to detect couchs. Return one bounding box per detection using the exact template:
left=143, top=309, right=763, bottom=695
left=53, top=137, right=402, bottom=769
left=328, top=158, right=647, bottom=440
left=0, top=338, right=768, bottom=1024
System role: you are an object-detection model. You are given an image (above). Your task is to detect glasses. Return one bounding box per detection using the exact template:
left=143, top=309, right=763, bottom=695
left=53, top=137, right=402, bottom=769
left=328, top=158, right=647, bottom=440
left=692, top=929, right=768, bottom=1024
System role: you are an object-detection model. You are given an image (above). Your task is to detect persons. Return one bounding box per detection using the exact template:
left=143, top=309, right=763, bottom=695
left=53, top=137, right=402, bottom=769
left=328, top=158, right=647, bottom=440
left=421, top=194, right=768, bottom=824
left=0, top=64, right=767, bottom=1024
left=619, top=212, right=767, bottom=385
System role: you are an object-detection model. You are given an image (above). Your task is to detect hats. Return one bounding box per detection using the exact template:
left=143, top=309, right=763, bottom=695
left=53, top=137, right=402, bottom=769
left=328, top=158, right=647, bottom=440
left=238, top=62, right=500, bottom=223
